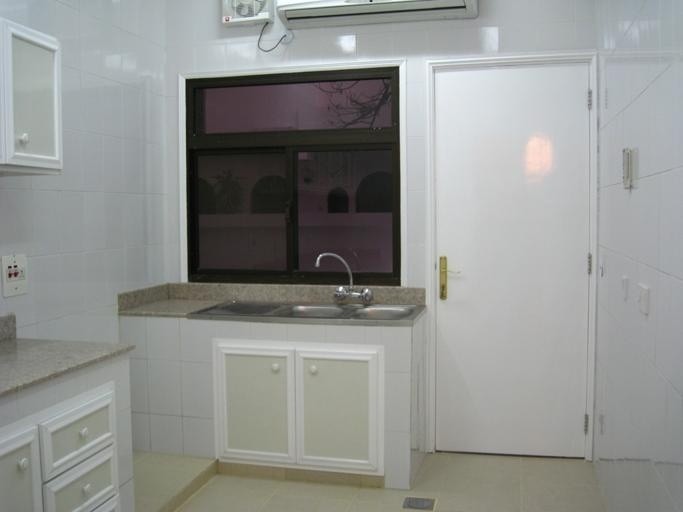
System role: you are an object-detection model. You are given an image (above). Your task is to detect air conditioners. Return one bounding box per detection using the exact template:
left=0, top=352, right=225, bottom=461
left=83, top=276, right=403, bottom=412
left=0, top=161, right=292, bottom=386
left=277, top=0, right=482, bottom=33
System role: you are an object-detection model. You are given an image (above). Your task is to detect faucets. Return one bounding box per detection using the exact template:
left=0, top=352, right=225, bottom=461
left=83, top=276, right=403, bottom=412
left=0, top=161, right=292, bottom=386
left=314, top=252, right=354, bottom=287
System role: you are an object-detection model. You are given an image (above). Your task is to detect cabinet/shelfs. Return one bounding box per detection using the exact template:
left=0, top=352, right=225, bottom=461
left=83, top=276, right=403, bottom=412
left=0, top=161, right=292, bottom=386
left=208, top=337, right=386, bottom=481
left=0, top=17, right=68, bottom=180
left=0, top=417, right=44, bottom=512
left=36, top=373, right=126, bottom=512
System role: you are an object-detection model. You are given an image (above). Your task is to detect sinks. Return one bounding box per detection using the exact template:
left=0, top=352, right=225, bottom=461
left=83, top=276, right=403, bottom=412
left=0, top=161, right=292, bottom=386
left=198, top=298, right=282, bottom=317
left=276, top=304, right=345, bottom=318
left=348, top=304, right=413, bottom=322
